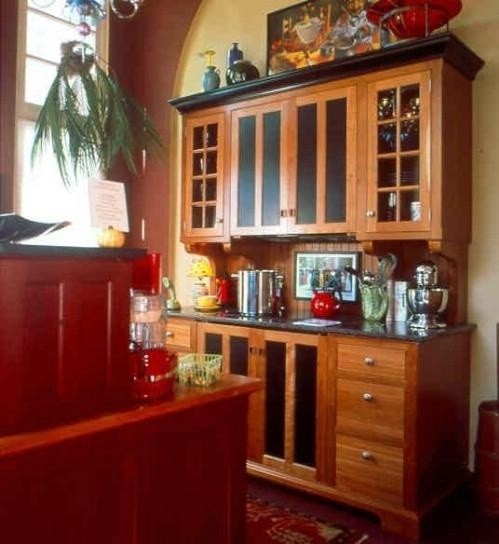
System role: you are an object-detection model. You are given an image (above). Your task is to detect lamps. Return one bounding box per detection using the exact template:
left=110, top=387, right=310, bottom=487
left=29, top=0, right=138, bottom=36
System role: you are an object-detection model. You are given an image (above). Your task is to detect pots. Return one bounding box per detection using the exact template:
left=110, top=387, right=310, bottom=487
left=407, top=259, right=450, bottom=329
left=231, top=266, right=283, bottom=316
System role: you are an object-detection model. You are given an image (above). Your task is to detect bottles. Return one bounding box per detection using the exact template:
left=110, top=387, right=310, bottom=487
left=228, top=41, right=243, bottom=66
left=381, top=191, right=396, bottom=221
left=202, top=64, right=219, bottom=90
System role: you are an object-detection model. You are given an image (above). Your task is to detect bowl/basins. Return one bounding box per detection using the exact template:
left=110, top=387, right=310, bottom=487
left=294, top=16, right=322, bottom=45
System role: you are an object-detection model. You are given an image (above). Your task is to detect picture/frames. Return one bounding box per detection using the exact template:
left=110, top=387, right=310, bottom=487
left=294, top=250, right=362, bottom=304
left=265, top=0, right=396, bottom=76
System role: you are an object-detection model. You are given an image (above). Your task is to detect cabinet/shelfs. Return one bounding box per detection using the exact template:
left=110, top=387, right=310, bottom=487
left=368, top=70, right=473, bottom=243
left=165, top=314, right=197, bottom=353
left=231, top=85, right=356, bottom=239
left=329, top=321, right=477, bottom=543
left=198, top=316, right=328, bottom=497
left=186, top=113, right=224, bottom=238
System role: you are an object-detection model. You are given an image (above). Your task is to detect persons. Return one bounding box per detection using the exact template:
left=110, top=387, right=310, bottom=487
left=299, top=268, right=352, bottom=291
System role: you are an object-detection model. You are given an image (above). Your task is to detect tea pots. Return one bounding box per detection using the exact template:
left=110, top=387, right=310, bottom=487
left=312, top=286, right=343, bottom=318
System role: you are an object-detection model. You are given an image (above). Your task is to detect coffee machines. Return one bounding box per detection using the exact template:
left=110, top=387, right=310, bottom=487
left=127, top=249, right=179, bottom=400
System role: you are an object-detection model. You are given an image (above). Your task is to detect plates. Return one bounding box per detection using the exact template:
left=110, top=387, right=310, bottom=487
left=0, top=210, right=71, bottom=245
left=195, top=305, right=219, bottom=311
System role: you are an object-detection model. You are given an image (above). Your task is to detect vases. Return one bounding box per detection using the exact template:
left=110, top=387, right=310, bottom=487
left=204, top=66, right=220, bottom=91
left=228, top=42, right=243, bottom=69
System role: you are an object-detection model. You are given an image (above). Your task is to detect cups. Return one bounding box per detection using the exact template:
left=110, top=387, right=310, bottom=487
left=198, top=296, right=217, bottom=306
left=362, top=320, right=384, bottom=336
left=358, top=280, right=388, bottom=320
left=410, top=201, right=421, bottom=220
